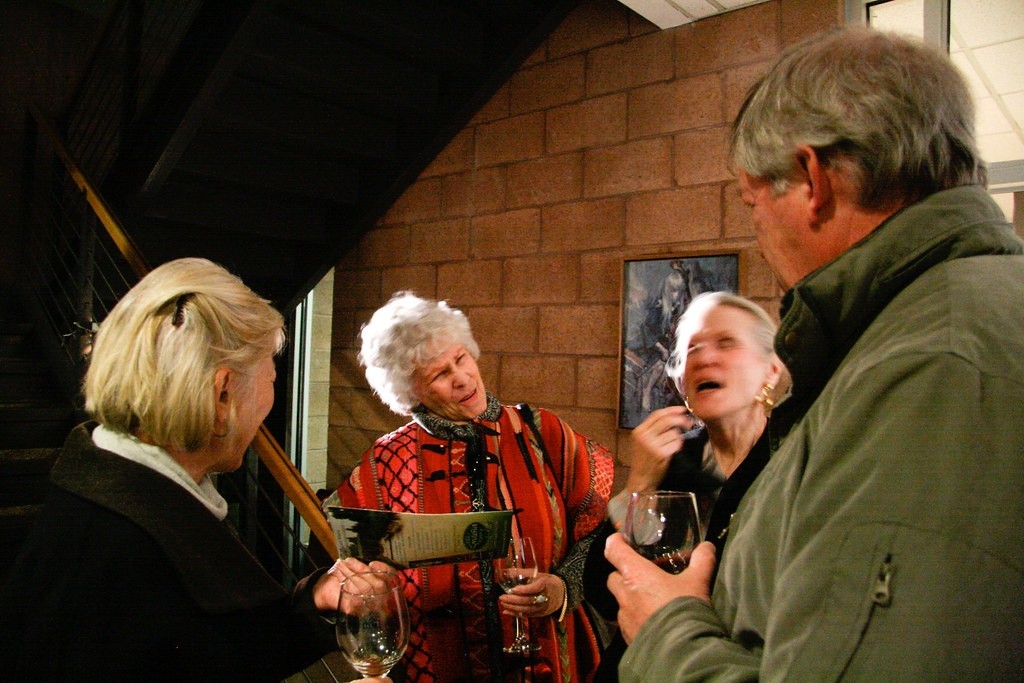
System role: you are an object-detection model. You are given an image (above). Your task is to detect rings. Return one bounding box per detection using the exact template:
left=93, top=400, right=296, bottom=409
left=533, top=594, right=550, bottom=606
left=519, top=612, right=523, bottom=618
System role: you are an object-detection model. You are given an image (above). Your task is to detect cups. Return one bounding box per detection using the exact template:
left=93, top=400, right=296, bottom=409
left=336, top=571, right=411, bottom=678
left=623, top=491, right=701, bottom=574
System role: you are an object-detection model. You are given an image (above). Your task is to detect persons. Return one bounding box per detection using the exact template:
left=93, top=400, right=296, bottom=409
left=0, top=256, right=390, bottom=683
left=299, top=288, right=628, bottom=683
left=583, top=290, right=793, bottom=683
left=604, top=26, right=1024, bottom=683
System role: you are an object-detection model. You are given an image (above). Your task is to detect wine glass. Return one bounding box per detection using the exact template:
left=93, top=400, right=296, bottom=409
left=496, top=538, right=542, bottom=652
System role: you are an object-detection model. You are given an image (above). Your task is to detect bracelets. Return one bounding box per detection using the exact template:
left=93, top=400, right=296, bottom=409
left=558, top=579, right=569, bottom=623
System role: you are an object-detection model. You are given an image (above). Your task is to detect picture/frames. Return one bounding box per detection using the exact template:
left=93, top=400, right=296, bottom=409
left=613, top=247, right=750, bottom=436
